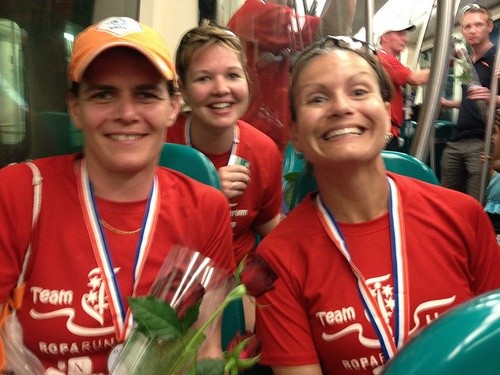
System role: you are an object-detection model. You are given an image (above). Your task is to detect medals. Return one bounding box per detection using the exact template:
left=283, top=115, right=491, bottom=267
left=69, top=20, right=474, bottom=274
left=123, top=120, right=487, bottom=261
left=107, top=344, right=125, bottom=374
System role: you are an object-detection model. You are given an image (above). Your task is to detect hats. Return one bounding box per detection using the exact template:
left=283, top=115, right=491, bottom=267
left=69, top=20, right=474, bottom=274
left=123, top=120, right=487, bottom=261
left=72, top=16, right=176, bottom=82
left=378, top=20, right=416, bottom=36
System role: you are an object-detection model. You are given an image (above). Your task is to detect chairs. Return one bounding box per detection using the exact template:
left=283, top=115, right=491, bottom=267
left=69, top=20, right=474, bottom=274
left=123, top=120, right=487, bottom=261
left=158, top=142, right=245, bottom=350
left=282, top=152, right=438, bottom=215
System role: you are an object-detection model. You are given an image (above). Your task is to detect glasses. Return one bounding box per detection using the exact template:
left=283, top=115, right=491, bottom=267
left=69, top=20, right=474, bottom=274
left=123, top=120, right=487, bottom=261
left=461, top=4, right=491, bottom=14
left=288, top=34, right=379, bottom=70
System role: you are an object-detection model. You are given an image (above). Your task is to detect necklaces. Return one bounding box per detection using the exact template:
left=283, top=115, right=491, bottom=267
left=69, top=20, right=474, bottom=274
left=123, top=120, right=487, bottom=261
left=97, top=214, right=143, bottom=238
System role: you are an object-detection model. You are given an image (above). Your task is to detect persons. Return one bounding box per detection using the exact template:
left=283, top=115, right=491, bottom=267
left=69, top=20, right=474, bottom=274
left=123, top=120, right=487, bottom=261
left=0, top=18, right=236, bottom=375
left=250, top=35, right=499, bottom=375
left=395, top=88, right=499, bottom=242
left=159, top=23, right=286, bottom=335
left=436, top=4, right=500, bottom=208
left=375, top=26, right=432, bottom=153
left=226, top=0, right=357, bottom=153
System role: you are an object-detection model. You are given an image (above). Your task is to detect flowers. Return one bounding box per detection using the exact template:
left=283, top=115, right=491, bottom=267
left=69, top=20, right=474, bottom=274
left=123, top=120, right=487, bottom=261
left=108, top=246, right=279, bottom=375
left=451, top=34, right=489, bottom=122
